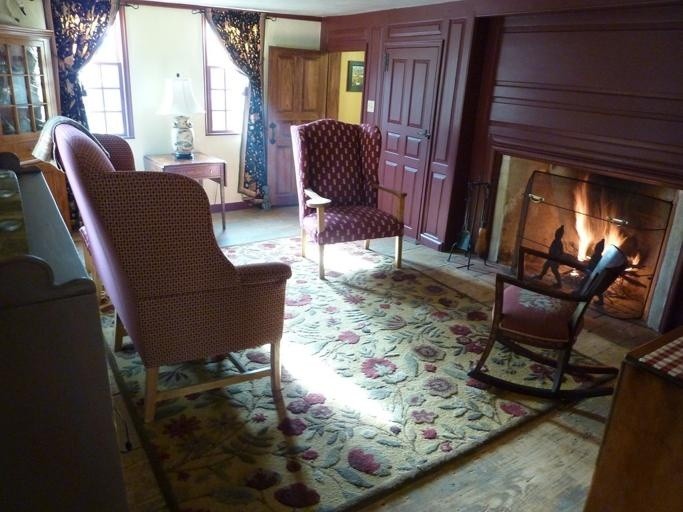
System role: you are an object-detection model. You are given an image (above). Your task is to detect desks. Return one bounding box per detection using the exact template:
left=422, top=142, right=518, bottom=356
left=585, top=325, right=683, bottom=512
left=143, top=151, right=227, bottom=231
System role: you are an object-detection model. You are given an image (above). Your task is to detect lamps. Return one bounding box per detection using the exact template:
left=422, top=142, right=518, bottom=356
left=154, top=80, right=205, bottom=155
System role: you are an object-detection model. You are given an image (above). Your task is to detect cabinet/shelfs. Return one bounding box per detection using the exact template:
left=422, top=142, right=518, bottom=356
left=0, top=25, right=72, bottom=240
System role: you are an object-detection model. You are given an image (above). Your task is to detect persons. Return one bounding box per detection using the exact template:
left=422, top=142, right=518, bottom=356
left=586, top=237, right=605, bottom=307
left=532, top=224, right=568, bottom=289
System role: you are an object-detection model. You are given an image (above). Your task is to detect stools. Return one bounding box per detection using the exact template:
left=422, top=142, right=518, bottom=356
left=78, top=224, right=111, bottom=305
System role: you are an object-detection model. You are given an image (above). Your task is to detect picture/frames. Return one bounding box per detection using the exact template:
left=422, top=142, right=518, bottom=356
left=346, top=60, right=365, bottom=92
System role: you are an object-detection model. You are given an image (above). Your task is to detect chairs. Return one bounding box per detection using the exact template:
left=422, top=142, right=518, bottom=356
left=51, top=115, right=292, bottom=424
left=466, top=240, right=629, bottom=404
left=290, top=117, right=409, bottom=278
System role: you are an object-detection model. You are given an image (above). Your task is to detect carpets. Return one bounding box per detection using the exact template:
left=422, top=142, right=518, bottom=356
left=89, top=234, right=618, bottom=512
